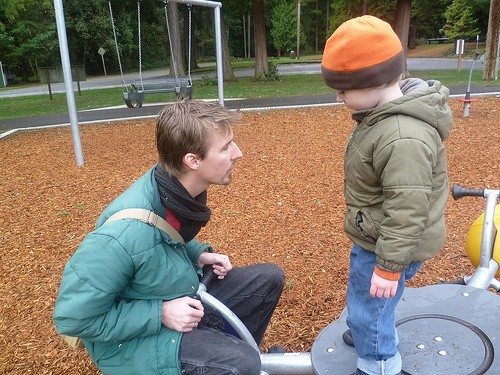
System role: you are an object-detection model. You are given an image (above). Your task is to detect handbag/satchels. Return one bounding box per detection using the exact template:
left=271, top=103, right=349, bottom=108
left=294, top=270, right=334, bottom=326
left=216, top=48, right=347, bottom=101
left=57, top=207, right=186, bottom=350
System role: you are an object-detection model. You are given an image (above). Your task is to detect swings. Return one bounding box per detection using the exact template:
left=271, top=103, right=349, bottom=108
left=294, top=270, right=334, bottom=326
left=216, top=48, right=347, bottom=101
left=109, top=0, right=145, bottom=109
left=164, top=2, right=193, bottom=101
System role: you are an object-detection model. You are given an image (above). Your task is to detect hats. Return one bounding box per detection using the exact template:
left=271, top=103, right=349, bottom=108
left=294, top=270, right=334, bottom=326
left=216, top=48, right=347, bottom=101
left=318, top=13, right=407, bottom=90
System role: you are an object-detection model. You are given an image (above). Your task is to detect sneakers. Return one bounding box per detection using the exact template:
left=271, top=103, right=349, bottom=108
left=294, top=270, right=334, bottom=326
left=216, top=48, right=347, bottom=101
left=342, top=330, right=355, bottom=348
left=351, top=369, right=368, bottom=375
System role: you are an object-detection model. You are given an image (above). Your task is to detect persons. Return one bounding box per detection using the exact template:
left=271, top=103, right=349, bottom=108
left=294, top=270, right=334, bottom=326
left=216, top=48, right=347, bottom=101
left=319, top=13, right=455, bottom=375
left=52, top=99, right=286, bottom=375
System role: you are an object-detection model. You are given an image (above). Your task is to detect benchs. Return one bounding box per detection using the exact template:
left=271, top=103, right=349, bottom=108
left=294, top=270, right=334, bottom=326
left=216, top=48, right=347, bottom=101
left=428, top=38, right=448, bottom=44
